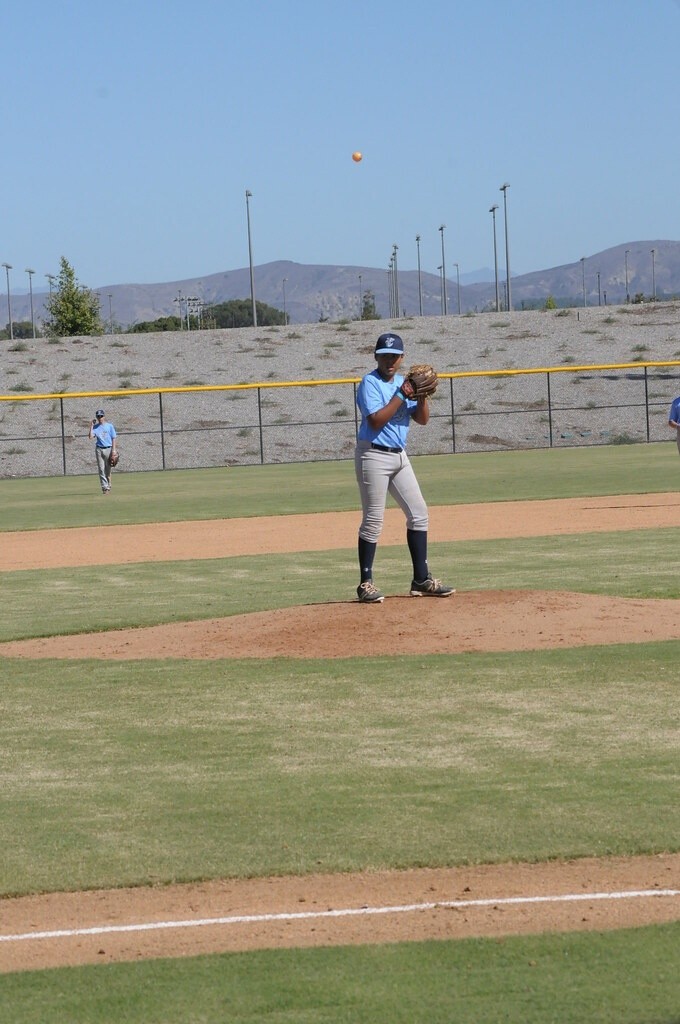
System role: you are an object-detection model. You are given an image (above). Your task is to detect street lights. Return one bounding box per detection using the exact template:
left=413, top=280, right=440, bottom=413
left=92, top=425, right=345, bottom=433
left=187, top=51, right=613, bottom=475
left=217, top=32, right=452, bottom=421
left=245, top=188, right=259, bottom=327
left=650, top=249, right=657, bottom=302
left=415, top=235, right=423, bottom=317
left=594, top=271, right=602, bottom=307
left=358, top=275, right=363, bottom=321
left=580, top=257, right=587, bottom=308
left=25, top=268, right=37, bottom=339
left=177, top=288, right=184, bottom=331
left=93, top=292, right=101, bottom=307
left=106, top=294, right=113, bottom=334
left=385, top=244, right=400, bottom=319
left=453, top=263, right=461, bottom=315
left=500, top=183, right=512, bottom=311
left=2, top=262, right=15, bottom=339
left=438, top=223, right=447, bottom=316
left=437, top=265, right=444, bottom=316
left=624, top=250, right=631, bottom=305
left=283, top=278, right=288, bottom=326
left=79, top=284, right=88, bottom=295
left=603, top=290, right=607, bottom=306
left=488, top=204, right=501, bottom=312
left=44, top=273, right=56, bottom=332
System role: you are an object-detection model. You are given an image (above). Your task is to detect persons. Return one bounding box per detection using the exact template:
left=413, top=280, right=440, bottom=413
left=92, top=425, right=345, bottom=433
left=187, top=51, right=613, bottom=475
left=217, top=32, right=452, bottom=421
left=669, top=396, right=680, bottom=456
left=355, top=333, right=456, bottom=602
left=89, top=410, right=117, bottom=494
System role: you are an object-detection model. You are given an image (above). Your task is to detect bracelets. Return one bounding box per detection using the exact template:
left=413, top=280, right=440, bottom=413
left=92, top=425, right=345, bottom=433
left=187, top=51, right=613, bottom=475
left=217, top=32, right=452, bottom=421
left=395, top=392, right=406, bottom=402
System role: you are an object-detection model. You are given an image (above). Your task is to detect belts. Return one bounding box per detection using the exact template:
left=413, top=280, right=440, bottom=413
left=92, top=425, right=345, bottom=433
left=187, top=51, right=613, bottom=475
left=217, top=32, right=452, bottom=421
left=371, top=443, right=403, bottom=453
left=97, top=445, right=111, bottom=449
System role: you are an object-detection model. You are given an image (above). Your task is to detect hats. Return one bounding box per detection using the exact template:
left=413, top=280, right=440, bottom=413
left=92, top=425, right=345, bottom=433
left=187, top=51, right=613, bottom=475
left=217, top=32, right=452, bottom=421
left=374, top=333, right=404, bottom=355
left=95, top=410, right=104, bottom=415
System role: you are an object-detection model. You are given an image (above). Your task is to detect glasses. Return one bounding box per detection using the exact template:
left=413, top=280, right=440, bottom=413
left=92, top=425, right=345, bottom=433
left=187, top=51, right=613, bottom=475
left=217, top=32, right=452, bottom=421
left=97, top=415, right=104, bottom=417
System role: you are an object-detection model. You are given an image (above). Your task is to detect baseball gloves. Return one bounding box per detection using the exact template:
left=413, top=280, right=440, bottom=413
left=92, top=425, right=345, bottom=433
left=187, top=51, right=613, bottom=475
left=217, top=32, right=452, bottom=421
left=398, top=365, right=438, bottom=401
left=109, top=450, right=119, bottom=467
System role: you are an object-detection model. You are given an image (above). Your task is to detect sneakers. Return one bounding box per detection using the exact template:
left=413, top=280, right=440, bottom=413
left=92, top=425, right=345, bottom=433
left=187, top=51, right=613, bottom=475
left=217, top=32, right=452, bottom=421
left=357, top=583, right=385, bottom=602
left=409, top=573, right=456, bottom=596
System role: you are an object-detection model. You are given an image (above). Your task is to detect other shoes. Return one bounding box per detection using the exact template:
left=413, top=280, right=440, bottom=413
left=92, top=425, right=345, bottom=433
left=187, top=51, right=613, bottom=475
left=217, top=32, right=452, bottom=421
left=103, top=488, right=110, bottom=494
